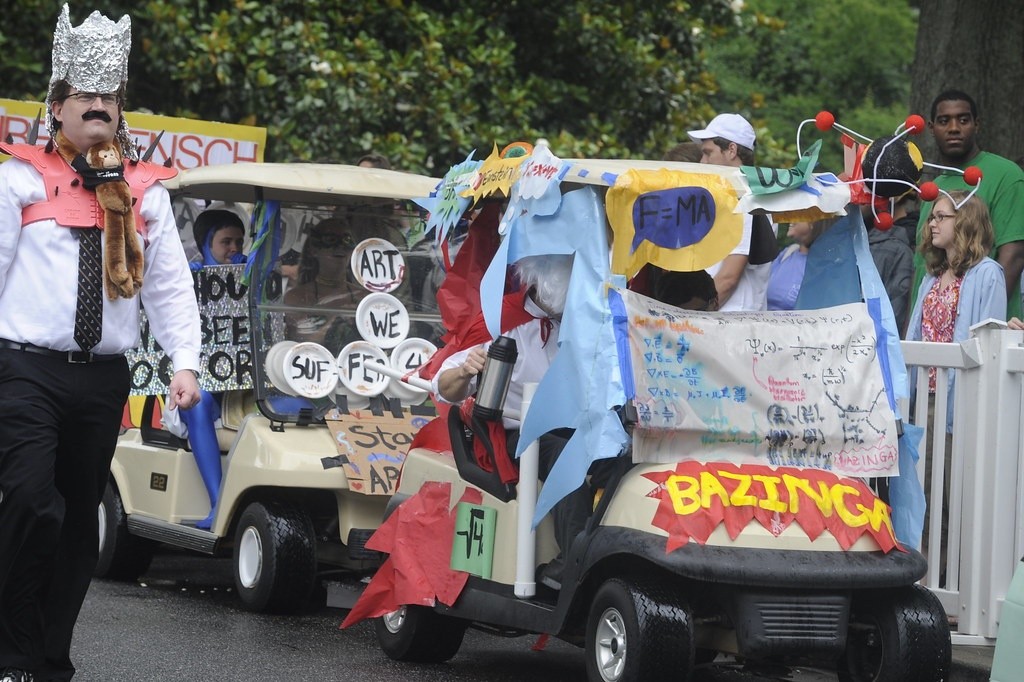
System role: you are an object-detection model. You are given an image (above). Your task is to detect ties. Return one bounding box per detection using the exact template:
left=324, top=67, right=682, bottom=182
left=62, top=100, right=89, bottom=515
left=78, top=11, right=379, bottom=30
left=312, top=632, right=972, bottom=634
left=74, top=225, right=102, bottom=354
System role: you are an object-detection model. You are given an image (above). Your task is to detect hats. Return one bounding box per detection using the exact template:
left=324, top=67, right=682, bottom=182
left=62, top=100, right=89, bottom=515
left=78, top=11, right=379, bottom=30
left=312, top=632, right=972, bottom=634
left=686, top=113, right=756, bottom=152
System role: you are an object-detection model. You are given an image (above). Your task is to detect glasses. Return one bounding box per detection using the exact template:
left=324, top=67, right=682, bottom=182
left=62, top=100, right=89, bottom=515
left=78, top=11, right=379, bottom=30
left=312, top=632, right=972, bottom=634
left=927, top=213, right=955, bottom=224
left=59, top=90, right=122, bottom=104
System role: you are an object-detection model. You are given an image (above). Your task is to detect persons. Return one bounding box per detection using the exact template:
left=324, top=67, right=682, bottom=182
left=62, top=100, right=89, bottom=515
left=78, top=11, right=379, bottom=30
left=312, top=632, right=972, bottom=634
left=0, top=76, right=202, bottom=682
left=432, top=252, right=617, bottom=605
left=909, top=90, right=1024, bottom=328
left=159, top=156, right=481, bottom=531
left=665, top=113, right=922, bottom=340
left=903, top=192, right=1008, bottom=557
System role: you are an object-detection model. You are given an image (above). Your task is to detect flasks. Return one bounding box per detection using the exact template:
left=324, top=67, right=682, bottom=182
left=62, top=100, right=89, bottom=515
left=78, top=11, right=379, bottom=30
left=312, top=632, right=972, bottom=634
left=473, top=335, right=518, bottom=423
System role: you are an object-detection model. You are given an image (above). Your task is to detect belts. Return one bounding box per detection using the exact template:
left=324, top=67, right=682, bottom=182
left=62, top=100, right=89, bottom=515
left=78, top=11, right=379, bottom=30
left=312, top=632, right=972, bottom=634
left=0, top=340, right=124, bottom=364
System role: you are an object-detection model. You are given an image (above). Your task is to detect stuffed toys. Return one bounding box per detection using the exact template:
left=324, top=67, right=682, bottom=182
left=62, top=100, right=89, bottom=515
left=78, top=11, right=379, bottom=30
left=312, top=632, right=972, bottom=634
left=55, top=128, right=143, bottom=300
